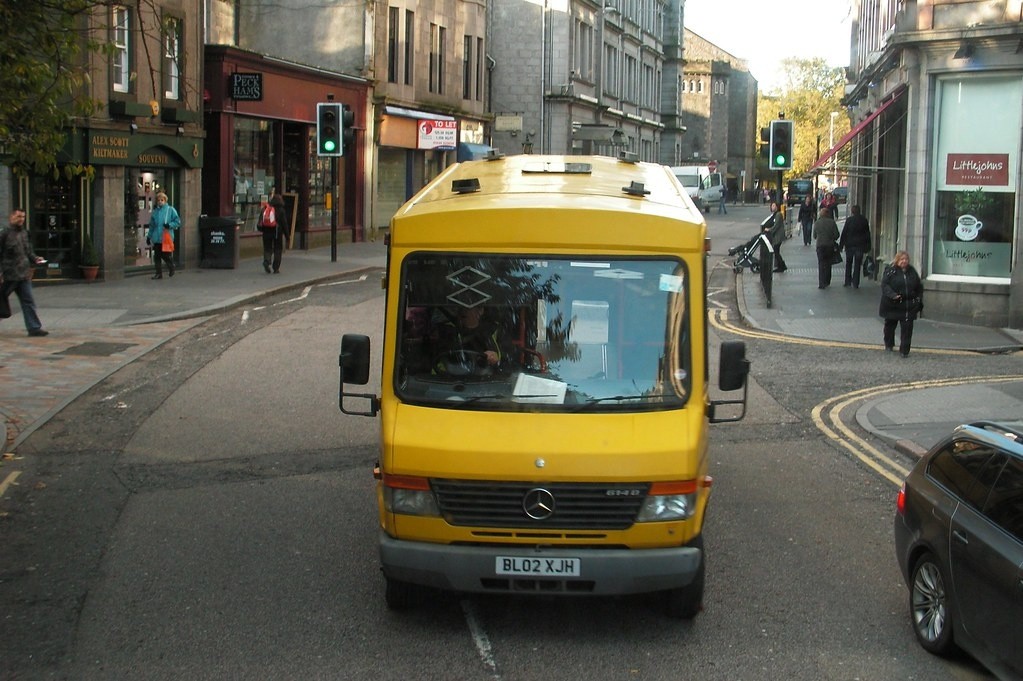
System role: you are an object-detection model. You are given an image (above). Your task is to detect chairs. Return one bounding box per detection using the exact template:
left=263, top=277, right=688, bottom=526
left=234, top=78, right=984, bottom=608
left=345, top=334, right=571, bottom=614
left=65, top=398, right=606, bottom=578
left=405, top=302, right=459, bottom=364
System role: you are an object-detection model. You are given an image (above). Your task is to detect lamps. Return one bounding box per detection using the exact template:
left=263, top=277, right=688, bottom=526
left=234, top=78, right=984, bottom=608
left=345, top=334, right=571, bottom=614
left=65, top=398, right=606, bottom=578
left=955, top=43, right=974, bottom=58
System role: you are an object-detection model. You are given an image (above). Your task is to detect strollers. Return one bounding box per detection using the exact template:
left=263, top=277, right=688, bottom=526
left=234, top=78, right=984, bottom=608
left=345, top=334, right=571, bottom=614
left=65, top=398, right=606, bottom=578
left=728, top=233, right=764, bottom=273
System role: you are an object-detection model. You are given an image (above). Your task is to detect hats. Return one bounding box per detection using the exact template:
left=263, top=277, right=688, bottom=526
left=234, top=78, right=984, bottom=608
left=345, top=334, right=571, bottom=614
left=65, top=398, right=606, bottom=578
left=157, top=193, right=168, bottom=202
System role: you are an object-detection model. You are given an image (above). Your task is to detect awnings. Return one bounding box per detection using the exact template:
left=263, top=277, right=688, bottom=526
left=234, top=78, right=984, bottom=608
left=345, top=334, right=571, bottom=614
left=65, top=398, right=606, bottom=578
left=808, top=88, right=908, bottom=177
left=572, top=127, right=629, bottom=145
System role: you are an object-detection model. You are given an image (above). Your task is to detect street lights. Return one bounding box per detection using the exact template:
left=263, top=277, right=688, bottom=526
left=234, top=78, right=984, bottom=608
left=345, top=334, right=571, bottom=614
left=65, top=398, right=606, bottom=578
left=830, top=111, right=839, bottom=149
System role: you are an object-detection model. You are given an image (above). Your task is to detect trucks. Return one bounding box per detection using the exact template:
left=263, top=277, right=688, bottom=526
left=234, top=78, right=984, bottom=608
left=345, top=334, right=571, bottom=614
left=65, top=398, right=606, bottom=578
left=670, top=165, right=722, bottom=212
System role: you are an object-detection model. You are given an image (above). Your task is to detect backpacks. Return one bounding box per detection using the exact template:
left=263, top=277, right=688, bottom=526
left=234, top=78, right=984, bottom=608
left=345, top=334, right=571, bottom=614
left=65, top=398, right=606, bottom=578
left=263, top=206, right=276, bottom=227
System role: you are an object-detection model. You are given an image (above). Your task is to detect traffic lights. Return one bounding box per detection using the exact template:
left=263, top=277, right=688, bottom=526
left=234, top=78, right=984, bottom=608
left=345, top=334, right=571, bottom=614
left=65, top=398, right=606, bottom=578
left=771, top=121, right=792, bottom=169
left=317, top=103, right=342, bottom=157
left=761, top=127, right=769, bottom=159
left=343, top=105, right=358, bottom=146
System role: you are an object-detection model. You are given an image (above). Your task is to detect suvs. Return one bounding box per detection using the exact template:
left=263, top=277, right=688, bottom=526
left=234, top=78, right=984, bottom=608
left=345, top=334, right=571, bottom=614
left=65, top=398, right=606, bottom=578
left=893, top=419, right=1023, bottom=681
left=786, top=179, right=813, bottom=208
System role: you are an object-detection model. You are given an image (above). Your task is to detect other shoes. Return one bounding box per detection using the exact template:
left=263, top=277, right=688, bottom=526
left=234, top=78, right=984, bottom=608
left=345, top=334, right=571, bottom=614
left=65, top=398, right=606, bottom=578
left=796, top=222, right=802, bottom=236
left=29, top=329, right=49, bottom=337
left=885, top=345, right=892, bottom=350
left=274, top=270, right=279, bottom=274
left=263, top=261, right=271, bottom=273
left=900, top=353, right=908, bottom=357
left=773, top=265, right=787, bottom=272
left=152, top=273, right=163, bottom=279
left=169, top=271, right=175, bottom=277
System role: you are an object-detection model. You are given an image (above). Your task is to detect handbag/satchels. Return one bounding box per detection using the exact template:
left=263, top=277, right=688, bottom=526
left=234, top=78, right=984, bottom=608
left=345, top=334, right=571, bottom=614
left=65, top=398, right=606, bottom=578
left=863, top=256, right=874, bottom=276
left=162, top=227, right=174, bottom=252
left=832, top=250, right=843, bottom=264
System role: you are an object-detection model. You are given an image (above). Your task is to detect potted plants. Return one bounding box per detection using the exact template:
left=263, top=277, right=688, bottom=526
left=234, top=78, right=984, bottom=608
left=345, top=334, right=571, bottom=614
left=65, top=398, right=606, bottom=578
left=79, top=234, right=100, bottom=280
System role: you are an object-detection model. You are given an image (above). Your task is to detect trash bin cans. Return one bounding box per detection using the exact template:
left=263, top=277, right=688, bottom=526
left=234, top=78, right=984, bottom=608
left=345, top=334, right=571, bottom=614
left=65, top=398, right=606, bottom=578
left=199, top=213, right=245, bottom=269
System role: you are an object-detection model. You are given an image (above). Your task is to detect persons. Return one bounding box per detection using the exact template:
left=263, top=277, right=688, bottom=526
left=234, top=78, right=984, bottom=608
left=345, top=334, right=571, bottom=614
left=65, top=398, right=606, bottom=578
left=256, top=194, right=289, bottom=274
left=764, top=201, right=787, bottom=272
left=0, top=208, right=47, bottom=336
left=425, top=289, right=516, bottom=374
left=798, top=194, right=816, bottom=246
left=717, top=183, right=727, bottom=215
left=840, top=205, right=870, bottom=289
left=761, top=186, right=784, bottom=204
left=813, top=208, right=838, bottom=289
left=879, top=251, right=923, bottom=358
left=819, top=193, right=838, bottom=220
left=146, top=193, right=180, bottom=280
left=731, top=182, right=738, bottom=204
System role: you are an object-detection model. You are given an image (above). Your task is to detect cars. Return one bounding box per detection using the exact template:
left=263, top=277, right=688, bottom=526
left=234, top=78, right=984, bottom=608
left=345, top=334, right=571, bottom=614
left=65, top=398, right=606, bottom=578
left=832, top=187, right=847, bottom=204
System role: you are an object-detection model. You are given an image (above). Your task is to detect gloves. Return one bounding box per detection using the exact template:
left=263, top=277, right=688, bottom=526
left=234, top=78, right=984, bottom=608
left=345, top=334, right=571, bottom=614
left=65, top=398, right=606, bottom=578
left=164, top=224, right=170, bottom=228
left=147, top=238, right=150, bottom=245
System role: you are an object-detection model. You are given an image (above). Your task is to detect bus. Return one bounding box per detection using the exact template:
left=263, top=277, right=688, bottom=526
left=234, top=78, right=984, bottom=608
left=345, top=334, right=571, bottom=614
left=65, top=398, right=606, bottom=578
left=336, top=154, right=750, bottom=610
